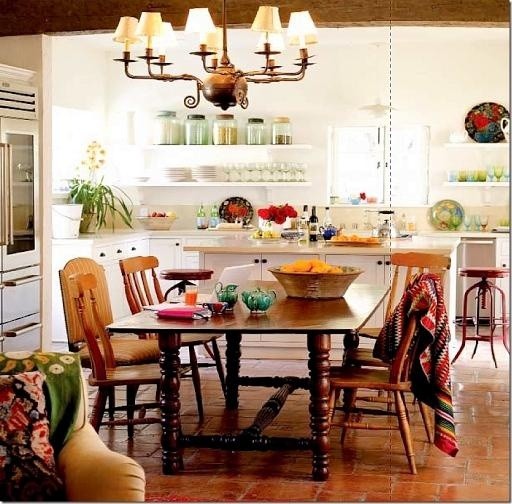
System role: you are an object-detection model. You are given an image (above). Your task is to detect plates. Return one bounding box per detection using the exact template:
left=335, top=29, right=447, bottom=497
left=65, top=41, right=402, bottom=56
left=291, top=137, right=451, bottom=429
left=219, top=197, right=254, bottom=226
left=326, top=238, right=383, bottom=247
left=249, top=238, right=287, bottom=244
left=159, top=165, right=219, bottom=183
left=465, top=102, right=510, bottom=143
left=429, top=200, right=465, bottom=230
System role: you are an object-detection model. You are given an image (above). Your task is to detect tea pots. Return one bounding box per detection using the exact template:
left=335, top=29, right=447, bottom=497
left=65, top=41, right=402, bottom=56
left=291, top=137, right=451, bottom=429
left=377, top=210, right=397, bottom=238
left=241, top=287, right=276, bottom=313
left=319, top=224, right=343, bottom=240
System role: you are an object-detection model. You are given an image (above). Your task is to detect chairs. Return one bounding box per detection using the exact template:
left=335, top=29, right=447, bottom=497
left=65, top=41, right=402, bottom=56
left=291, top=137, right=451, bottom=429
left=59, top=257, right=159, bottom=435
left=357, top=252, right=458, bottom=420
left=0, top=352, right=147, bottom=502
left=69, top=272, right=192, bottom=437
left=330, top=273, right=459, bottom=475
left=117, top=256, right=227, bottom=422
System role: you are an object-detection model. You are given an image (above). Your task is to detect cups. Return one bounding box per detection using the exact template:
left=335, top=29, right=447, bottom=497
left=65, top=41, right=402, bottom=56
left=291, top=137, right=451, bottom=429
left=202, top=302, right=228, bottom=314
left=405, top=214, right=416, bottom=231
left=447, top=167, right=487, bottom=182
left=185, top=285, right=197, bottom=306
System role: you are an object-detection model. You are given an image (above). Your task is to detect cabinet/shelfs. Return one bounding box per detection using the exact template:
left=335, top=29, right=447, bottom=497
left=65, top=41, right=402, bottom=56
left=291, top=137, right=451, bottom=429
left=51, top=233, right=151, bottom=344
left=183, top=236, right=461, bottom=360
left=327, top=125, right=430, bottom=209
left=133, top=143, right=311, bottom=230
left=0, top=62, right=41, bottom=356
left=443, top=141, right=511, bottom=231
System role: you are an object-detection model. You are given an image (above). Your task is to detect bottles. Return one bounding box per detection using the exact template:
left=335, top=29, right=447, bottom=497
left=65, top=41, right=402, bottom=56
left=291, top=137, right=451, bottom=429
left=196, top=201, right=206, bottom=229
left=301, top=205, right=332, bottom=241
left=154, top=110, right=181, bottom=145
left=212, top=114, right=237, bottom=145
left=183, top=114, right=208, bottom=145
left=244, top=118, right=266, bottom=145
left=211, top=201, right=219, bottom=227
left=271, top=117, right=292, bottom=144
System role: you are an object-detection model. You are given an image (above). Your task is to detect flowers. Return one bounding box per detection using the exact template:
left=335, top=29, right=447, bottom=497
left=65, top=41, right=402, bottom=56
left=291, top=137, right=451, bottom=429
left=58, top=140, right=133, bottom=228
left=257, top=202, right=297, bottom=223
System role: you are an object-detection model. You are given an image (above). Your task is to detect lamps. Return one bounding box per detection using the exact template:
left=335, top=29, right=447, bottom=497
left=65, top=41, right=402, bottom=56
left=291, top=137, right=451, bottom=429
left=112, top=0, right=318, bottom=110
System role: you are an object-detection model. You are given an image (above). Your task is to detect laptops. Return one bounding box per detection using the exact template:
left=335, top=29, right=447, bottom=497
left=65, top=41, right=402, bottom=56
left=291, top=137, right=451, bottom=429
left=169, top=263, right=256, bottom=304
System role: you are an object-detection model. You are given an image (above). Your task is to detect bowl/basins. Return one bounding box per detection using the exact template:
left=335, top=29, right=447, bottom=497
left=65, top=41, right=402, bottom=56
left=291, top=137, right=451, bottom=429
left=136, top=176, right=150, bottom=182
left=281, top=232, right=304, bottom=242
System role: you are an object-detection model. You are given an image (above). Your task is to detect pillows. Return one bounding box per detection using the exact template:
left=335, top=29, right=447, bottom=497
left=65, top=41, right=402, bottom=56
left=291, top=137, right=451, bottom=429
left=0, top=371, right=66, bottom=501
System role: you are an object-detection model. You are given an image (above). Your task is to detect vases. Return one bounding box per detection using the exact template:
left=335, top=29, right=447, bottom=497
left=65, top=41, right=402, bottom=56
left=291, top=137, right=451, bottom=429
left=259, top=218, right=292, bottom=236
left=79, top=213, right=97, bottom=233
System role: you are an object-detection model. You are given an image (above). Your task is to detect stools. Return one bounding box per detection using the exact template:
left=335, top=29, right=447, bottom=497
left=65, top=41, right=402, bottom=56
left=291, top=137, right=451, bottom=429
left=160, top=269, right=225, bottom=368
left=451, top=267, right=510, bottom=366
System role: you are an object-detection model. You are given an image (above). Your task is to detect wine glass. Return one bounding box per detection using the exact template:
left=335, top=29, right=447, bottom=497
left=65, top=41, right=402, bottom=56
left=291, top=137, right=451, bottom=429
left=463, top=215, right=488, bottom=232
left=487, top=165, right=509, bottom=182
left=223, top=162, right=305, bottom=182
